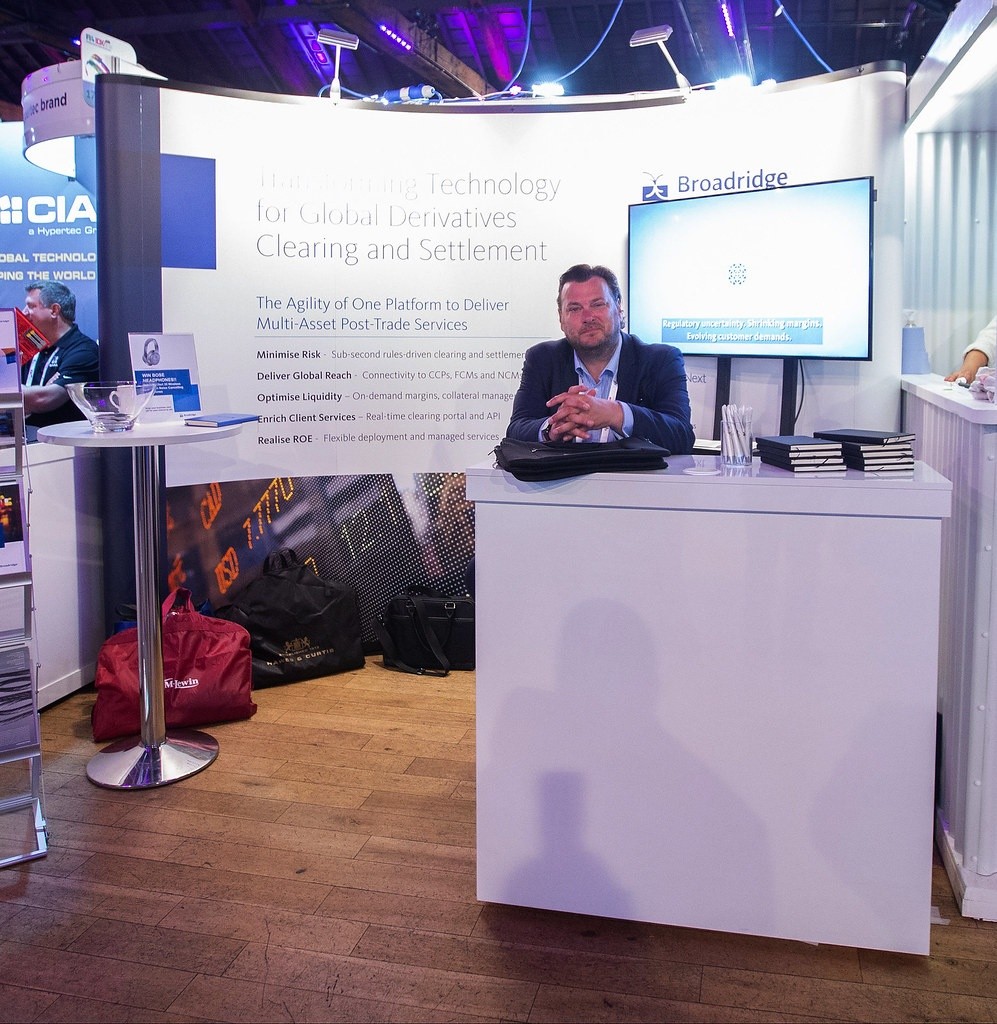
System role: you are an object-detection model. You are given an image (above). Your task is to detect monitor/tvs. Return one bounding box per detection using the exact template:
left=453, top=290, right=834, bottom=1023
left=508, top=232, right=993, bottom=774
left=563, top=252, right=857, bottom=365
left=627, top=176, right=876, bottom=360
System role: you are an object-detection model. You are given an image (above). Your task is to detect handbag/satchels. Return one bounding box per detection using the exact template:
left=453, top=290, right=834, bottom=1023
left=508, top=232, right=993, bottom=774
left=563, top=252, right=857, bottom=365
left=90, top=587, right=258, bottom=741
left=373, top=585, right=476, bottom=676
left=212, top=547, right=367, bottom=688
left=494, top=434, right=669, bottom=481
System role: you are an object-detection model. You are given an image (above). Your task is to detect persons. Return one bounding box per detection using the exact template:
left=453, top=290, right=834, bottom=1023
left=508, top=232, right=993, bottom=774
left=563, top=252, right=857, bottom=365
left=464, top=264, right=696, bottom=601
left=21, top=279, right=100, bottom=428
left=944, top=315, right=997, bottom=384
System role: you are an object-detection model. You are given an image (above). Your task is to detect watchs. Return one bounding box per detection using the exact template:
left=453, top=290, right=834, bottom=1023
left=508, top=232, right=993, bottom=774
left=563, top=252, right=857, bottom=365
left=541, top=424, right=552, bottom=442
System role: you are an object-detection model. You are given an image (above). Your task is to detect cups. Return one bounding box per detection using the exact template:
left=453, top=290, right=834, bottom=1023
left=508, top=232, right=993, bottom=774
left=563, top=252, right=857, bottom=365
left=110, top=385, right=136, bottom=414
left=720, top=421, right=752, bottom=466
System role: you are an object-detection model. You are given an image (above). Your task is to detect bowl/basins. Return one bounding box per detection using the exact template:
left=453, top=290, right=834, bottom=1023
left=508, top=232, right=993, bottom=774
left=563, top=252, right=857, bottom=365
left=66, top=380, right=157, bottom=433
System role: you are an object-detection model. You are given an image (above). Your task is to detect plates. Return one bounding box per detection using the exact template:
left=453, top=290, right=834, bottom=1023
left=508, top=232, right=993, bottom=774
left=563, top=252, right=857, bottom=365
left=682, top=467, right=721, bottom=476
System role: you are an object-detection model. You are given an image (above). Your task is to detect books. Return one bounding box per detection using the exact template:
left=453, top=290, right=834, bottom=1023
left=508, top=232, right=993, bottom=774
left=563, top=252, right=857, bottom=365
left=755, top=436, right=848, bottom=472
left=0, top=641, right=38, bottom=752
left=0, top=472, right=30, bottom=576
left=184, top=414, right=259, bottom=428
left=813, top=429, right=916, bottom=472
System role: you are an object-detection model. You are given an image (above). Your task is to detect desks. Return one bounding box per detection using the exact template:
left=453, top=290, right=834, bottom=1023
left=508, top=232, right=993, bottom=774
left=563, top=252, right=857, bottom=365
left=34, top=409, right=251, bottom=797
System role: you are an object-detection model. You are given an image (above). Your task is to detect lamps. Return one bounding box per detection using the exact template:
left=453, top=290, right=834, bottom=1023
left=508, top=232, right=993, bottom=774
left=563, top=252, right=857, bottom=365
left=629, top=24, right=693, bottom=94
left=313, top=27, right=364, bottom=104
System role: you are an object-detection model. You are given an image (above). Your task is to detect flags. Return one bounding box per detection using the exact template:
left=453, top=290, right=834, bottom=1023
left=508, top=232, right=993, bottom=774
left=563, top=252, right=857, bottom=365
left=15, top=307, right=50, bottom=366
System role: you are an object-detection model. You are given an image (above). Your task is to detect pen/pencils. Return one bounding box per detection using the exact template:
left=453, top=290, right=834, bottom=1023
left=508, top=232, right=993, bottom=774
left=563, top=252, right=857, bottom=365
left=721, top=404, right=753, bottom=465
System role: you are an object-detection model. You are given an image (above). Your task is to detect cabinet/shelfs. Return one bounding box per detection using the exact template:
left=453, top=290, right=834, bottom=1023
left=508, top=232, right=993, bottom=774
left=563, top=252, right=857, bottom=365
left=467, top=445, right=956, bottom=953
left=0, top=308, right=51, bottom=880
